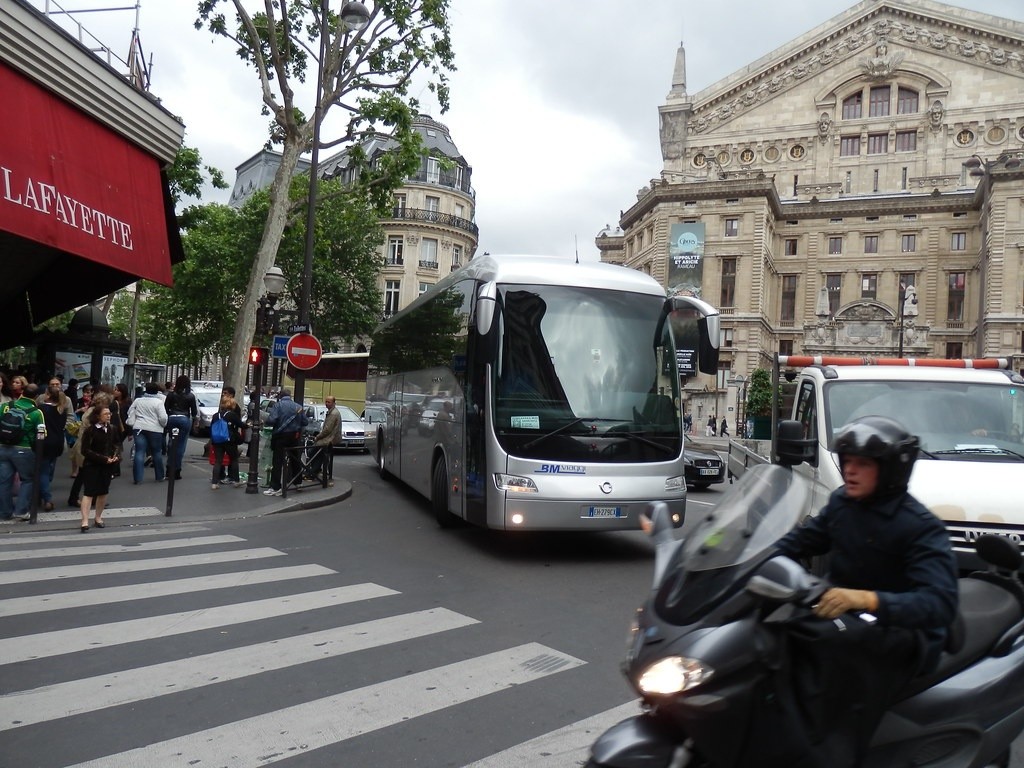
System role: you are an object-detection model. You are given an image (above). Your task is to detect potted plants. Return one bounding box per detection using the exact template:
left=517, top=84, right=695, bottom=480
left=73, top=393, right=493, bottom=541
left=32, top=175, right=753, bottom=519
left=745, top=368, right=783, bottom=439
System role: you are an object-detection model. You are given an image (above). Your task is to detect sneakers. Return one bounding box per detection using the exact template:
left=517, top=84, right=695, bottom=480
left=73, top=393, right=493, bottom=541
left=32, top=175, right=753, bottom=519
left=263, top=487, right=282, bottom=496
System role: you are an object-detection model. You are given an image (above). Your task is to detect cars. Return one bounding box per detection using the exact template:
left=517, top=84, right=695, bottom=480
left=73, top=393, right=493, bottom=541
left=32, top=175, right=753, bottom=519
left=190, top=380, right=282, bottom=439
left=682, top=434, right=726, bottom=491
left=305, top=404, right=365, bottom=450
left=365, top=390, right=464, bottom=449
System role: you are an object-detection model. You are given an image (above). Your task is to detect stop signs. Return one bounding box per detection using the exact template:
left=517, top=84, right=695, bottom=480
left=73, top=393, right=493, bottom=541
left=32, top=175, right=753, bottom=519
left=287, top=332, right=323, bottom=369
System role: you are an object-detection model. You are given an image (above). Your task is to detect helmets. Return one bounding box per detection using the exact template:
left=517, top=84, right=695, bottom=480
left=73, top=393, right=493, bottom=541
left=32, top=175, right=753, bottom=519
left=830, top=415, right=921, bottom=495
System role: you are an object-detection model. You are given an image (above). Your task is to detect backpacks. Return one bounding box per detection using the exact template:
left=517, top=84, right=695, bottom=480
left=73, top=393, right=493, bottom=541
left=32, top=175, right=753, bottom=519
left=0, top=401, right=38, bottom=445
left=210, top=411, right=231, bottom=445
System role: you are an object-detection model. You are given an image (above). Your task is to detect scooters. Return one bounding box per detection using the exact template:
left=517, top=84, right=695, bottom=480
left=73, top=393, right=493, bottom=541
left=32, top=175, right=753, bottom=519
left=584, top=458, right=1024, bottom=768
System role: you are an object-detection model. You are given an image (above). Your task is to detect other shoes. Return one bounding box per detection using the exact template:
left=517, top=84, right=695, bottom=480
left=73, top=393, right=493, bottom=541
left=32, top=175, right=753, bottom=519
left=209, top=478, right=247, bottom=489
left=71, top=473, right=77, bottom=478
left=92, top=501, right=109, bottom=506
left=12, top=512, right=31, bottom=520
left=286, top=483, right=303, bottom=489
left=144, top=456, right=152, bottom=466
left=302, top=468, right=322, bottom=480
left=158, top=477, right=165, bottom=482
left=43, top=503, right=54, bottom=511
left=135, top=480, right=144, bottom=485
left=68, top=499, right=81, bottom=507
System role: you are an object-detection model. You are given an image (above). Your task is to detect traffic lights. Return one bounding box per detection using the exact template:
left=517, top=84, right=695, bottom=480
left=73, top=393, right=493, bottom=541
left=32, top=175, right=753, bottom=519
left=249, top=348, right=269, bottom=366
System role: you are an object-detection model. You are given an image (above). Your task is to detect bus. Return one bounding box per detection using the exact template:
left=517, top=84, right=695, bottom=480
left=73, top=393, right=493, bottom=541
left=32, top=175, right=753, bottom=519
left=282, top=352, right=369, bottom=418
left=365, top=250, right=723, bottom=534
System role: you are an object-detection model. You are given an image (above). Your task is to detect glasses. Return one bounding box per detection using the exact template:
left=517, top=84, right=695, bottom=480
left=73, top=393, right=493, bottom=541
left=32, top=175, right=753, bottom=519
left=103, top=413, right=113, bottom=416
left=222, top=391, right=228, bottom=394
left=115, top=389, right=120, bottom=392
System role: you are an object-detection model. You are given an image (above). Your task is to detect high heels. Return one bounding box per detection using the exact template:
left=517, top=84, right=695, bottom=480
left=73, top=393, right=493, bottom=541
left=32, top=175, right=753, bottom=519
left=94, top=518, right=105, bottom=528
left=81, top=525, right=89, bottom=533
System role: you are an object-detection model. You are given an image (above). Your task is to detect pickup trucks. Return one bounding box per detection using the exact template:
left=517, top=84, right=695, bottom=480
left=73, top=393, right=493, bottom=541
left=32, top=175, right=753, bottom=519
left=726, top=352, right=1024, bottom=562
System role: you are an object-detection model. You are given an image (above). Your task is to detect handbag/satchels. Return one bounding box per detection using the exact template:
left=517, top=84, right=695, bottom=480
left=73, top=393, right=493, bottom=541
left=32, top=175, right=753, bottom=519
left=58, top=431, right=64, bottom=456
left=125, top=398, right=138, bottom=425
left=115, top=400, right=124, bottom=432
left=271, top=432, right=279, bottom=451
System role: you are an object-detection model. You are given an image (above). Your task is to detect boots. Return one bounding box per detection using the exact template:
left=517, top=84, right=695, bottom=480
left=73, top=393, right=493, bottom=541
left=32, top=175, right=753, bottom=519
left=166, top=465, right=182, bottom=480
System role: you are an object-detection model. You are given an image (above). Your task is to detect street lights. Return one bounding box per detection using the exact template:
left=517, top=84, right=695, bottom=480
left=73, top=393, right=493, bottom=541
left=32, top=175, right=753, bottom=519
left=292, top=0, right=371, bottom=407
left=897, top=282, right=918, bottom=358
left=246, top=266, right=287, bottom=496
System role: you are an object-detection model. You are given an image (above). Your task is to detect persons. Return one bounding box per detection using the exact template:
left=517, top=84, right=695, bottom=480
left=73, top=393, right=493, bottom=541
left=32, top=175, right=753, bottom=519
left=705, top=414, right=716, bottom=438
left=720, top=416, right=730, bottom=438
left=772, top=415, right=960, bottom=767
left=259, top=388, right=266, bottom=396
left=962, top=389, right=1011, bottom=441
left=683, top=412, right=693, bottom=434
left=0, top=362, right=198, bottom=532
left=262, top=389, right=342, bottom=496
left=210, top=387, right=253, bottom=489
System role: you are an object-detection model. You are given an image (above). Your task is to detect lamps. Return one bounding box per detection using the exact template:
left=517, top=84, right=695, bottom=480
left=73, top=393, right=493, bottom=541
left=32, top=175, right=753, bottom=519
left=963, top=152, right=1020, bottom=181
